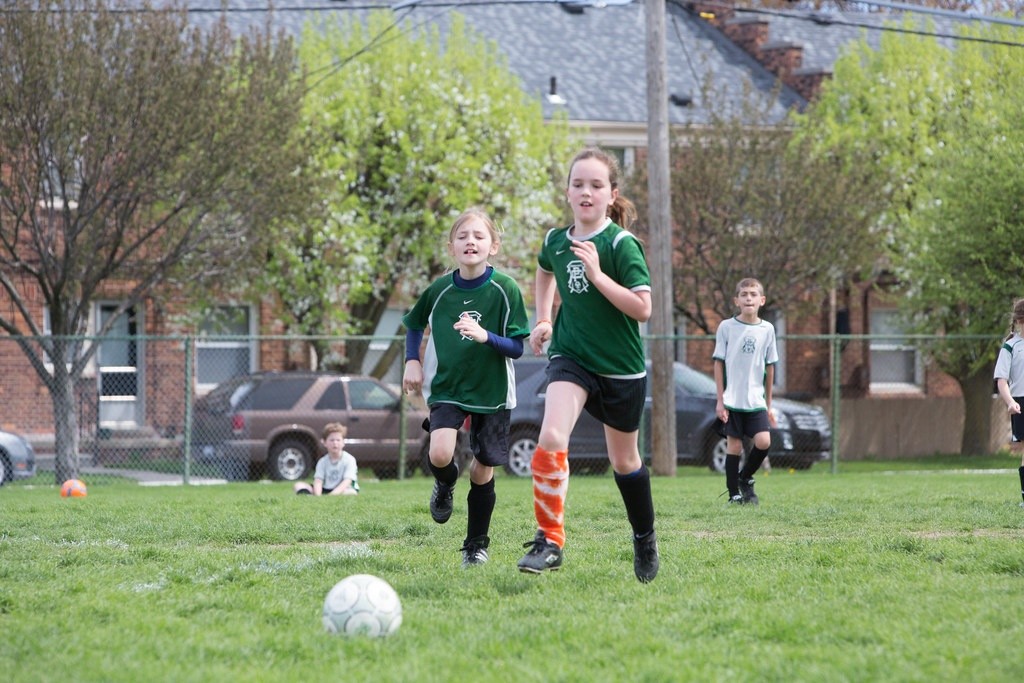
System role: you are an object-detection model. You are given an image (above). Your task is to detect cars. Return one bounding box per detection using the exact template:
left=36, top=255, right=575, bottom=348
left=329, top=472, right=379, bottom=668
left=504, top=354, right=833, bottom=479
left=0, top=430, right=35, bottom=486
left=189, top=368, right=466, bottom=483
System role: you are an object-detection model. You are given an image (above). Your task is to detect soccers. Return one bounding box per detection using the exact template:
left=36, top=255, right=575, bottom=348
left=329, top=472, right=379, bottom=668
left=321, top=573, right=403, bottom=637
left=60, top=479, right=87, bottom=498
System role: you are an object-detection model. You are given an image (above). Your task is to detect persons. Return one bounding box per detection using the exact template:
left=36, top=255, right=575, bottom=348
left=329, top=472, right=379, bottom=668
left=294, top=422, right=359, bottom=495
left=517, top=148, right=660, bottom=585
left=401, top=212, right=530, bottom=571
left=993, top=299, right=1024, bottom=506
left=711, top=278, right=780, bottom=505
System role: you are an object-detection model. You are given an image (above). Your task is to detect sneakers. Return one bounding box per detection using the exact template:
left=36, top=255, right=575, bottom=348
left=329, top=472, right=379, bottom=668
left=430, top=463, right=459, bottom=524
left=634, top=529, right=658, bottom=584
left=517, top=529, right=562, bottom=573
left=460, top=536, right=490, bottom=573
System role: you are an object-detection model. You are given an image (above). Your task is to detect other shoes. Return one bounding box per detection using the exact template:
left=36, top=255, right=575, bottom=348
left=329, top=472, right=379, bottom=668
left=738, top=475, right=758, bottom=506
left=728, top=494, right=743, bottom=507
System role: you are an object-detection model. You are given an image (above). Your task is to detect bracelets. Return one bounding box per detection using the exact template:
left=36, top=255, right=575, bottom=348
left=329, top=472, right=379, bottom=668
left=536, top=320, right=551, bottom=325
